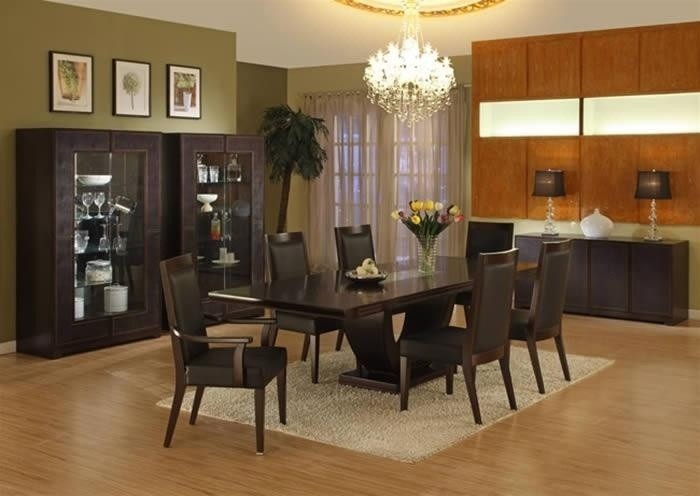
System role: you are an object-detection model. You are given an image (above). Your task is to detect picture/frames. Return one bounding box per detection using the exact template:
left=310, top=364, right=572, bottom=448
left=166, top=64, right=201, bottom=119
left=48, top=51, right=93, bottom=114
left=112, top=59, right=152, bottom=116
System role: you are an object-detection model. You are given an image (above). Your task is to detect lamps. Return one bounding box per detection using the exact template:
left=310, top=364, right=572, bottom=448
left=363, top=0, right=457, bottom=129
left=531, top=169, right=566, bottom=236
left=634, top=168, right=672, bottom=242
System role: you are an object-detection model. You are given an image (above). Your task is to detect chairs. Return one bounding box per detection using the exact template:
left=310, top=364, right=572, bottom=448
left=159, top=251, right=287, bottom=455
left=512, top=240, right=572, bottom=394
left=262, top=231, right=344, bottom=384
left=398, top=247, right=517, bottom=425
left=335, top=225, right=376, bottom=351
left=455, top=221, right=514, bottom=324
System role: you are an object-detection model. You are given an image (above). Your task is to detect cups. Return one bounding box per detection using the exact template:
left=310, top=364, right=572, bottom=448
left=219, top=248, right=227, bottom=261
left=74, top=230, right=89, bottom=255
left=208, top=165, right=219, bottom=183
left=198, top=166, right=208, bottom=183
left=227, top=253, right=234, bottom=262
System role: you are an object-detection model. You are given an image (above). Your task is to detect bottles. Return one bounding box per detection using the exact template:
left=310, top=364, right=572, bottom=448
left=211, top=213, right=221, bottom=241
left=221, top=212, right=233, bottom=239
left=228, top=152, right=243, bottom=182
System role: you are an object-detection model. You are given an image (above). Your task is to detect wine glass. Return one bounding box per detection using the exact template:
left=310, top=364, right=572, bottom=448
left=93, top=192, right=106, bottom=219
left=81, top=192, right=94, bottom=219
left=113, top=223, right=123, bottom=249
left=98, top=224, right=111, bottom=251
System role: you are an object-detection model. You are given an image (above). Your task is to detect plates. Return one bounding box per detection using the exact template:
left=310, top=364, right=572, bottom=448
left=76, top=175, right=113, bottom=186
left=344, top=269, right=388, bottom=284
left=75, top=298, right=85, bottom=320
left=212, top=260, right=240, bottom=265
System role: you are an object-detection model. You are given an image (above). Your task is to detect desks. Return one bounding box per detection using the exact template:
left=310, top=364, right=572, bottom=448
left=208, top=254, right=538, bottom=395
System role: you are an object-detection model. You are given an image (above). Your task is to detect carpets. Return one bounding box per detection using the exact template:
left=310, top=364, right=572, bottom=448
left=156, top=344, right=616, bottom=464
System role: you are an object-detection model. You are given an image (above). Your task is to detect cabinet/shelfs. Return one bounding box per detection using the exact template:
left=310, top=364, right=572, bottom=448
left=516, top=236, right=589, bottom=316
left=588, top=240, right=628, bottom=321
left=629, top=243, right=673, bottom=327
left=169, top=132, right=265, bottom=327
left=15, top=129, right=163, bottom=359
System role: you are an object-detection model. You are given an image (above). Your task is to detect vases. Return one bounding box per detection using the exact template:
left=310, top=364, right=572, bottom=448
left=413, top=236, right=438, bottom=274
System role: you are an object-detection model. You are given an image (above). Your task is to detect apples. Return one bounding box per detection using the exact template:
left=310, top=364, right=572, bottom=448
left=361, top=257, right=375, bottom=271
left=366, top=266, right=379, bottom=277
left=355, top=265, right=367, bottom=277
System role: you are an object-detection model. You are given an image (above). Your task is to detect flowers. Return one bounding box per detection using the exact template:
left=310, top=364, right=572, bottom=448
left=390, top=198, right=465, bottom=259
